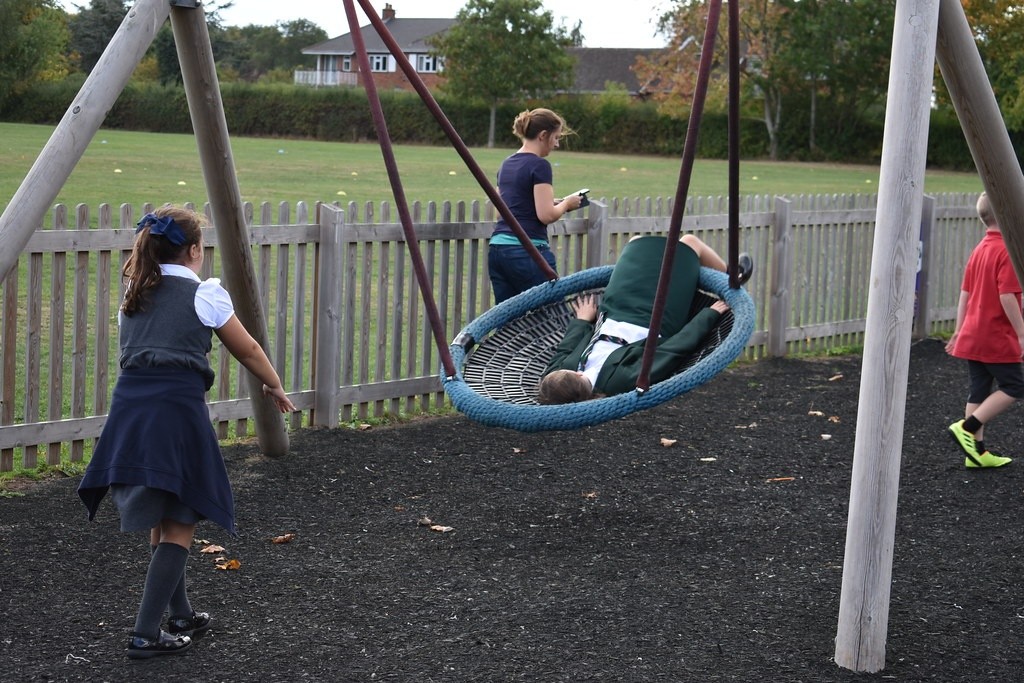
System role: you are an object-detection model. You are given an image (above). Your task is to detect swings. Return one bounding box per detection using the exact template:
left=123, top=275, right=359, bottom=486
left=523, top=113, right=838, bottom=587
left=340, top=0, right=762, bottom=436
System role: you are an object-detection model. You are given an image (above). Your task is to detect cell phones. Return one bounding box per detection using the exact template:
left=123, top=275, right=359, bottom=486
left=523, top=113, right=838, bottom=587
left=563, top=188, right=590, bottom=200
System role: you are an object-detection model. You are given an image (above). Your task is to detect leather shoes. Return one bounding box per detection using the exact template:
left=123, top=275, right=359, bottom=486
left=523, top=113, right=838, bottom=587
left=738, top=253, right=753, bottom=286
left=168, top=609, right=210, bottom=635
left=126, top=627, right=192, bottom=658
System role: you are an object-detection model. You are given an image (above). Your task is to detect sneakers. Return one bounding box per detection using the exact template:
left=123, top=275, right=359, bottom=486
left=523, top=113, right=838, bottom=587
left=965, top=448, right=1013, bottom=468
left=948, top=419, right=983, bottom=466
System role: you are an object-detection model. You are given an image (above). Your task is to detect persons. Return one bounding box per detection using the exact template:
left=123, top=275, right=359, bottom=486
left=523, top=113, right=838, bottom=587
left=109, top=207, right=298, bottom=657
left=944, top=189, right=1024, bottom=470
left=486, top=108, right=584, bottom=306
left=536, top=234, right=754, bottom=404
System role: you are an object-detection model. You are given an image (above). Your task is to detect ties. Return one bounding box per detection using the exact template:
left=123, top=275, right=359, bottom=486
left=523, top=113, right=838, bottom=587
left=579, top=311, right=608, bottom=371
